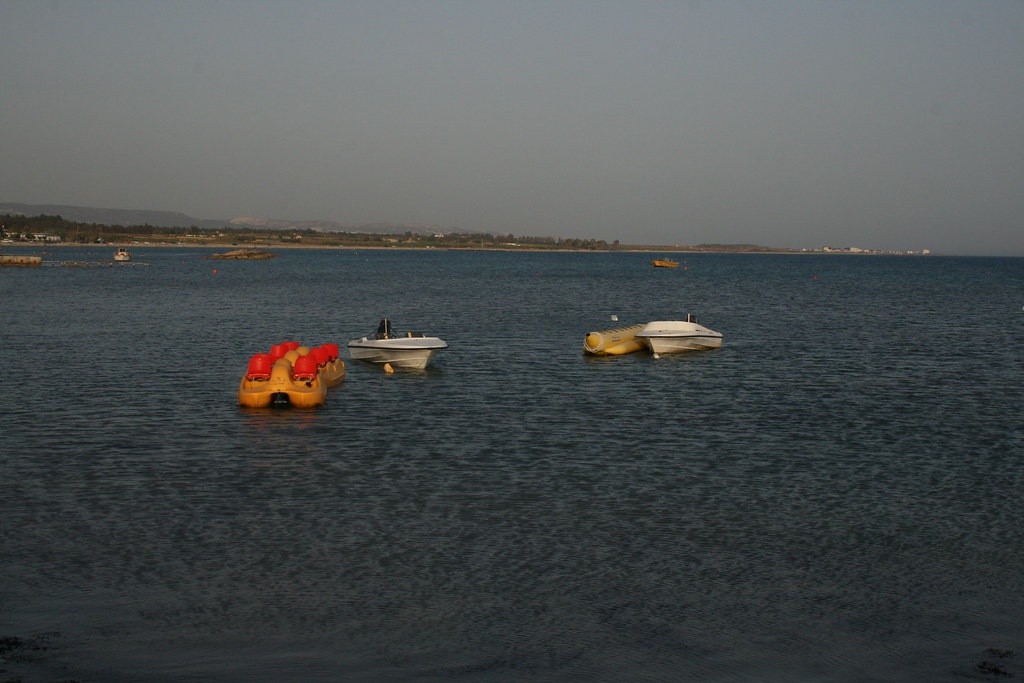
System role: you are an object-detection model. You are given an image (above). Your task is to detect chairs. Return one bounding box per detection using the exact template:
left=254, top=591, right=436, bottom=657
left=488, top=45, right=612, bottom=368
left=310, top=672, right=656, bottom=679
left=282, top=341, right=298, bottom=353
left=270, top=345, right=286, bottom=365
left=309, top=348, right=328, bottom=367
left=247, top=353, right=271, bottom=381
left=375, top=320, right=391, bottom=336
left=293, top=355, right=316, bottom=380
left=322, top=344, right=339, bottom=363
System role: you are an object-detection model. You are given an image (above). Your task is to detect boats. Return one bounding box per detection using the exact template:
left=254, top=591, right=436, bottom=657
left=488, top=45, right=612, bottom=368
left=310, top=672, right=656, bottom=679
left=653, top=258, right=681, bottom=270
left=348, top=318, right=450, bottom=371
left=241, top=340, right=347, bottom=412
left=633, top=311, right=724, bottom=358
left=113, top=248, right=131, bottom=261
